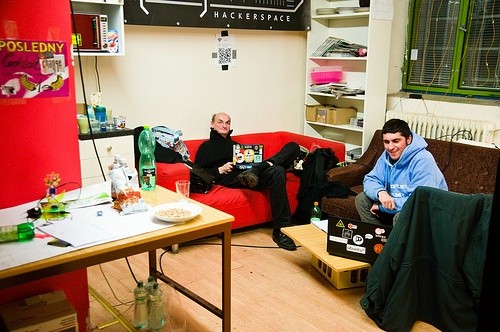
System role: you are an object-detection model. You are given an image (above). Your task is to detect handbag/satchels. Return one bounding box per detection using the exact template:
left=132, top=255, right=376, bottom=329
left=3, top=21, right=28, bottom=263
left=189, top=165, right=216, bottom=193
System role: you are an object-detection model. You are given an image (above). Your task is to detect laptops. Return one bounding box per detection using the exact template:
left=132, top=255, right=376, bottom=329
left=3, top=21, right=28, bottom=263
left=232, top=144, right=264, bottom=169
left=327, top=216, right=394, bottom=264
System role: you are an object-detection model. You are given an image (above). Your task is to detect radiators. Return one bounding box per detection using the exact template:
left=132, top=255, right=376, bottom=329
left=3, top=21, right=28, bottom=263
left=386, top=109, right=493, bottom=147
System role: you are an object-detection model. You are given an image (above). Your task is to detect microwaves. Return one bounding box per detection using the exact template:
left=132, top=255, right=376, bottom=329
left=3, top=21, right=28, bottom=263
left=72, top=13, right=108, bottom=52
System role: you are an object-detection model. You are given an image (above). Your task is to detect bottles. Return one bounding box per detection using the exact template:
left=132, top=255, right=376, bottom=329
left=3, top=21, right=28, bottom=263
left=0, top=222, right=35, bottom=243
left=149, top=283, right=164, bottom=329
left=95, top=106, right=106, bottom=123
left=134, top=282, right=147, bottom=329
left=138, top=125, right=156, bottom=191
left=311, top=202, right=321, bottom=224
left=144, top=277, right=155, bottom=322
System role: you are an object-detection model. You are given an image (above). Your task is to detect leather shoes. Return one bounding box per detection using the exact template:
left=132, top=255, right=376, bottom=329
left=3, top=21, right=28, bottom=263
left=238, top=170, right=258, bottom=186
left=272, top=232, right=296, bottom=249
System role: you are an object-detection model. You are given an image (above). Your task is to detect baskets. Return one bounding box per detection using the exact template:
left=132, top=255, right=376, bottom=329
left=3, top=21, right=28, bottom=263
left=311, top=71, right=343, bottom=83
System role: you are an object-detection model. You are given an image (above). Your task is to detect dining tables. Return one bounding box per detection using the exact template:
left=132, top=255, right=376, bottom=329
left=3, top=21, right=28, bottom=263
left=0, top=182, right=235, bottom=332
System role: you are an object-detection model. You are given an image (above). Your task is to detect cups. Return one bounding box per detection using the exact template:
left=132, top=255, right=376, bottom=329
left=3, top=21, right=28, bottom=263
left=176, top=180, right=190, bottom=204
left=114, top=117, right=126, bottom=129
left=100, top=123, right=106, bottom=132
left=91, top=121, right=99, bottom=132
left=77, top=118, right=89, bottom=133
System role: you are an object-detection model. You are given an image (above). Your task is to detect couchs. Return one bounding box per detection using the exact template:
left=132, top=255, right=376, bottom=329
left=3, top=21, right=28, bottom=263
left=319, top=130, right=500, bottom=233
left=154, top=131, right=346, bottom=253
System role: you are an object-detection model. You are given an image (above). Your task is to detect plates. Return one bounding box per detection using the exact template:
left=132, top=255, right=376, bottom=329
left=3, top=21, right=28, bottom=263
left=336, top=7, right=357, bottom=14
left=154, top=203, right=202, bottom=222
left=316, top=7, right=336, bottom=14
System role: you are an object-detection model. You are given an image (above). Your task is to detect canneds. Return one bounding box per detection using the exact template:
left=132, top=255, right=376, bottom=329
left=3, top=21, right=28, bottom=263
left=107, top=29, right=118, bottom=53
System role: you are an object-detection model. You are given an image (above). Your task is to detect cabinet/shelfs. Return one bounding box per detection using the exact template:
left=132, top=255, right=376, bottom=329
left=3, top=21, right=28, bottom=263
left=79, top=127, right=136, bottom=186
left=304, top=0, right=395, bottom=162
left=70, top=0, right=124, bottom=57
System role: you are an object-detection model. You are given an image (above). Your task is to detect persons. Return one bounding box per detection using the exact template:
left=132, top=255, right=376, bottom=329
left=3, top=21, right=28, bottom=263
left=195, top=113, right=300, bottom=251
left=354, top=119, right=448, bottom=227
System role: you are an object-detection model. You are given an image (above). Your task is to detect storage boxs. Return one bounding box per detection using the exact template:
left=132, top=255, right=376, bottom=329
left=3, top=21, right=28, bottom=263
left=306, top=104, right=357, bottom=124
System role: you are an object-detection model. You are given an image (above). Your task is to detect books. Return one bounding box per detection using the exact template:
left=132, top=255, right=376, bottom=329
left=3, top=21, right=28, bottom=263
left=312, top=37, right=366, bottom=57
left=311, top=84, right=363, bottom=99
